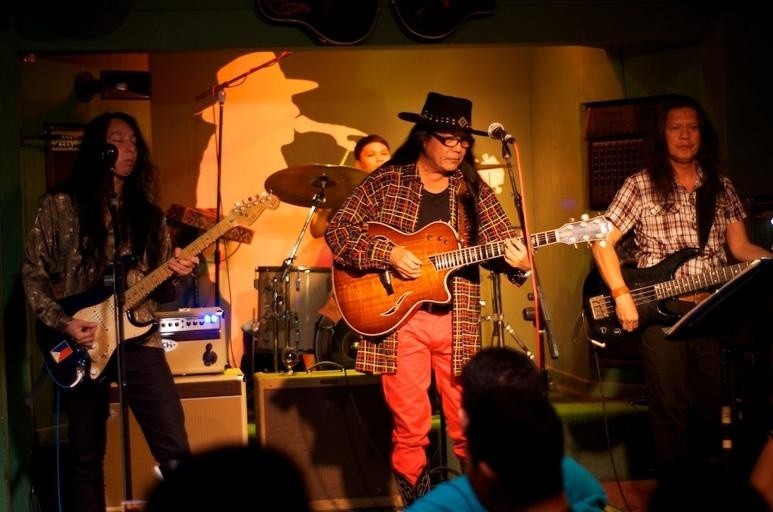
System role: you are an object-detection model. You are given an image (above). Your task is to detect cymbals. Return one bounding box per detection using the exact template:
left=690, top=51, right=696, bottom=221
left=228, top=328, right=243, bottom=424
left=264, top=164, right=371, bottom=208
left=473, top=162, right=517, bottom=169
left=510, top=225, right=524, bottom=229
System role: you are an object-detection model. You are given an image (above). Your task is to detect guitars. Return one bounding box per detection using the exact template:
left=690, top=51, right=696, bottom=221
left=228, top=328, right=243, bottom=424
left=583, top=247, right=754, bottom=346
left=332, top=215, right=612, bottom=337
left=37, top=188, right=280, bottom=390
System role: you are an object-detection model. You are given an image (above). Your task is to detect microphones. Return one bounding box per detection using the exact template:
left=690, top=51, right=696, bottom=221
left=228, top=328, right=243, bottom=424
left=93, top=144, right=123, bottom=180
left=485, top=120, right=516, bottom=148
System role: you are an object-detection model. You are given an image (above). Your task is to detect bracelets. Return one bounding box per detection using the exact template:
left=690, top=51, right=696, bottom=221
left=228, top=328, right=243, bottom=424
left=610, top=285, right=629, bottom=298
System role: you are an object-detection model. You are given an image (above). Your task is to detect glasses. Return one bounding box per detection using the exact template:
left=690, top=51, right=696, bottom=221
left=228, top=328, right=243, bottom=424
left=432, top=132, right=474, bottom=148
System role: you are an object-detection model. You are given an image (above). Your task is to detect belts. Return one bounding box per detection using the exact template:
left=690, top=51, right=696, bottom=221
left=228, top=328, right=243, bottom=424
left=420, top=302, right=453, bottom=316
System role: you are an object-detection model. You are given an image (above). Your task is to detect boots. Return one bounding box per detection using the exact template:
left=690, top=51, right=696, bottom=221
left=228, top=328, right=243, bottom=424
left=393, top=467, right=430, bottom=507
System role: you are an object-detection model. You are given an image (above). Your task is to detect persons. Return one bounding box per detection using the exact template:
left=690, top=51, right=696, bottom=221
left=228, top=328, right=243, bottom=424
left=462, top=388, right=574, bottom=512
left=401, top=346, right=608, bottom=512
left=21, top=111, right=201, bottom=511
left=143, top=443, right=310, bottom=511
left=590, top=95, right=773, bottom=482
left=309, top=135, right=394, bottom=330
left=644, top=465, right=773, bottom=509
left=323, top=91, right=536, bottom=511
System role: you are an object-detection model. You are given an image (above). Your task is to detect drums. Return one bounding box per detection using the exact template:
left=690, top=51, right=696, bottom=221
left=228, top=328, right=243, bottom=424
left=256, top=266, right=331, bottom=352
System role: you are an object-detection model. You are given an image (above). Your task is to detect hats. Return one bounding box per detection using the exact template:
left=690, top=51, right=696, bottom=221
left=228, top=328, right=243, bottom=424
left=398, top=92, right=489, bottom=136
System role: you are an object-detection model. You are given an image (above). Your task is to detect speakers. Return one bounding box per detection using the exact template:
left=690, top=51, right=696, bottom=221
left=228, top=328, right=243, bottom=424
left=253, top=369, right=449, bottom=512
left=100, top=368, right=250, bottom=507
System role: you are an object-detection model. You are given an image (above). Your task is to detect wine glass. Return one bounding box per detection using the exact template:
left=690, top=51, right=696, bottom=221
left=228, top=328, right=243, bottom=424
left=281, top=347, right=299, bottom=375
